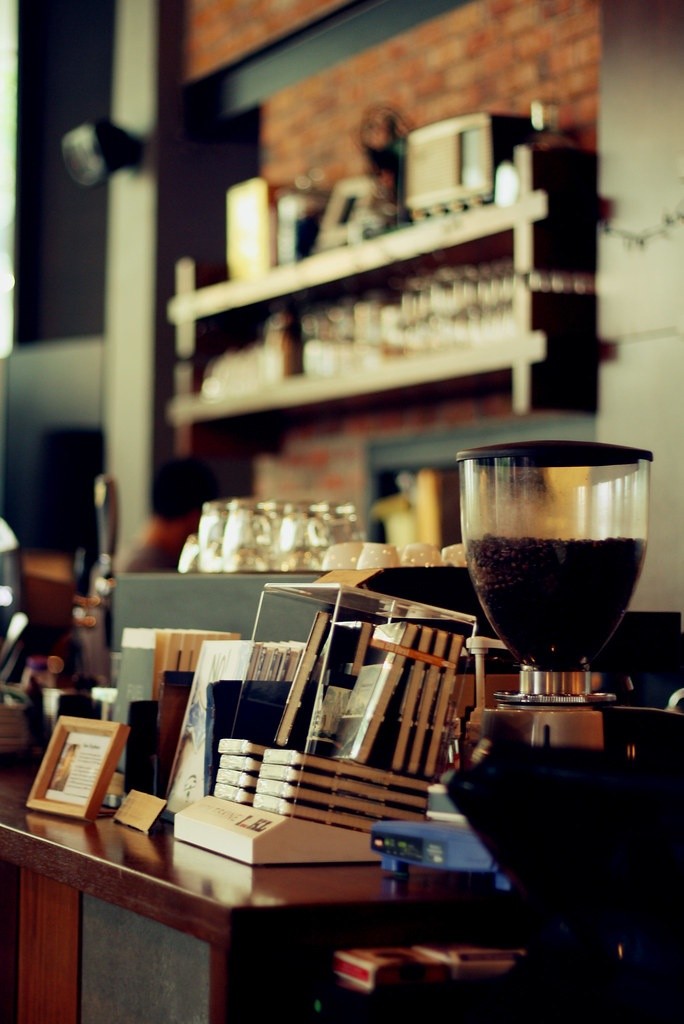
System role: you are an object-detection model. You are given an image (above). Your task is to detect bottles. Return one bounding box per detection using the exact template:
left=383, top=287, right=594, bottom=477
left=198, top=501, right=358, bottom=574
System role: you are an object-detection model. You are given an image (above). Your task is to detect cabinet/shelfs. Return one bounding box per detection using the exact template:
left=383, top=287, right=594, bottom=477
left=166, top=147, right=613, bottom=454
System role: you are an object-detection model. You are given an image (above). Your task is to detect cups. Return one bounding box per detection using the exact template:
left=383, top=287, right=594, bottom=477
left=322, top=542, right=468, bottom=571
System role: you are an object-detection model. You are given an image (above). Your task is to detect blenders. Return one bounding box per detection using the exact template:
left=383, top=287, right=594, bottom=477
left=459, top=444, right=653, bottom=753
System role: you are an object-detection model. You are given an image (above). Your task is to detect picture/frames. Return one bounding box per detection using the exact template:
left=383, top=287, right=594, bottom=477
left=27, top=715, right=130, bottom=824
